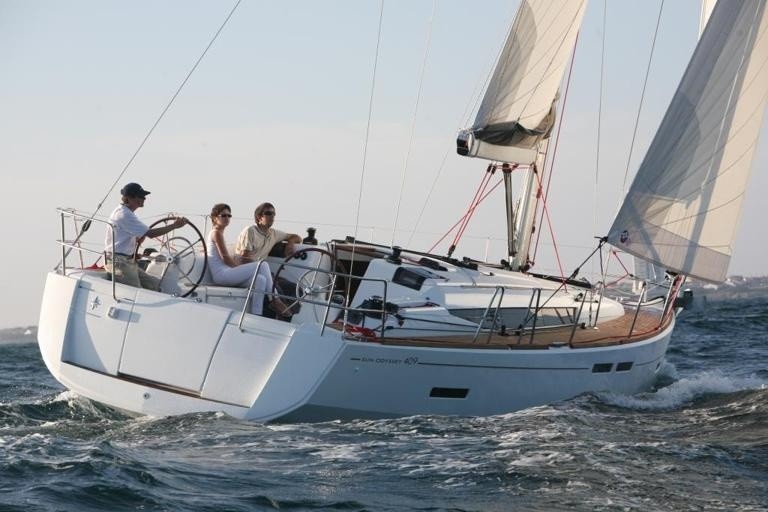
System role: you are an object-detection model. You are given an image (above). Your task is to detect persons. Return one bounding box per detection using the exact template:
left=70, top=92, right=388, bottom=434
left=206, top=203, right=292, bottom=321
left=235, top=203, right=301, bottom=264
left=104, top=182, right=188, bottom=287
left=303, top=228, right=317, bottom=245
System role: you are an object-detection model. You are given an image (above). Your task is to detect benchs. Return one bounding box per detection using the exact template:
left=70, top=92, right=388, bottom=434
left=180, top=254, right=254, bottom=314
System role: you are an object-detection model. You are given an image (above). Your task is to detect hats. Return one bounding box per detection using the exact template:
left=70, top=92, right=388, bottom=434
left=120, top=182, right=151, bottom=196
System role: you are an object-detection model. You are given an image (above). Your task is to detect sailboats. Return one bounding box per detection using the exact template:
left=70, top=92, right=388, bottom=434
left=37, top=1, right=768, bottom=418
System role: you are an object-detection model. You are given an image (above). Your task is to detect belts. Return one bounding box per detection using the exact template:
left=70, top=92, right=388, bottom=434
left=106, top=250, right=134, bottom=259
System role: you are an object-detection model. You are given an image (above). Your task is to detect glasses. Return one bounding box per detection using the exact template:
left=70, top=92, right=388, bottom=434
left=134, top=194, right=147, bottom=202
left=256, top=211, right=276, bottom=216
left=213, top=213, right=234, bottom=219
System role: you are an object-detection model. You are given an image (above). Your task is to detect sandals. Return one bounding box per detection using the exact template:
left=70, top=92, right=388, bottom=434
left=268, top=302, right=292, bottom=318
